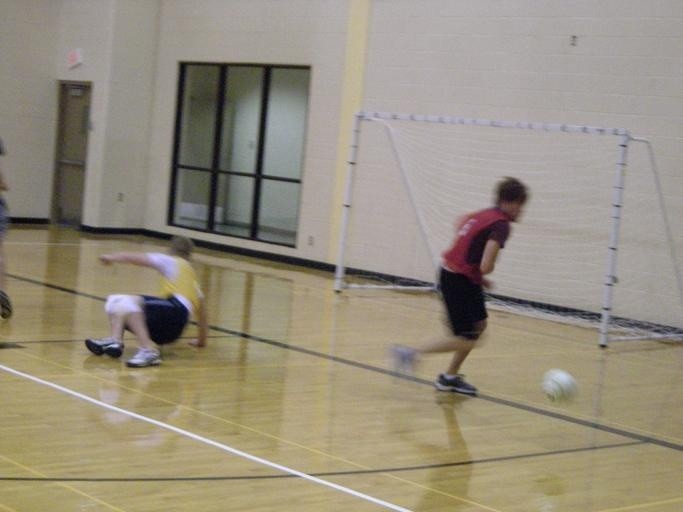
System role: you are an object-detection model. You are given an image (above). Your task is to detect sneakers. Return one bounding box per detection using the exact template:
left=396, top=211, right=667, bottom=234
left=435, top=373, right=477, bottom=395
left=0, top=291, right=11, bottom=319
left=126, top=348, right=163, bottom=368
left=84, top=337, right=124, bottom=358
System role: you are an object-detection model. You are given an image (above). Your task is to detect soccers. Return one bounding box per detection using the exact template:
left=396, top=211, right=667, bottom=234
left=541, top=369, right=577, bottom=402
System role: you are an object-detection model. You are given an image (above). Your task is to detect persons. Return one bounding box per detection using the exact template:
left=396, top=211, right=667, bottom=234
left=395, top=179, right=527, bottom=394
left=0, top=139, right=20, bottom=347
left=85, top=236, right=208, bottom=368
